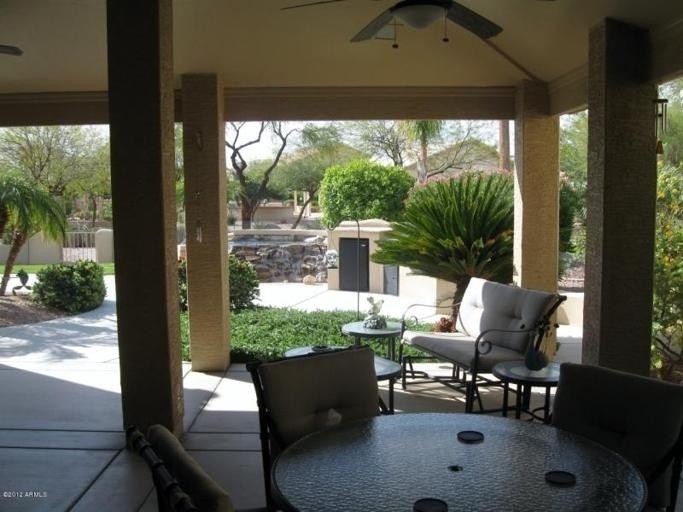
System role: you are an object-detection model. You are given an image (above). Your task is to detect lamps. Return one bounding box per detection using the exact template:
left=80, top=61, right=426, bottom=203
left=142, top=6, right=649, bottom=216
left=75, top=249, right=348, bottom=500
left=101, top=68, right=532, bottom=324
left=388, top=2, right=452, bottom=32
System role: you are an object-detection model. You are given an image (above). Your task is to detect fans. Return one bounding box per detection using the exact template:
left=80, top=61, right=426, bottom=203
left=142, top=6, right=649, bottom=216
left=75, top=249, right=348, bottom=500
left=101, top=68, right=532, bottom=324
left=277, top=0, right=504, bottom=50
